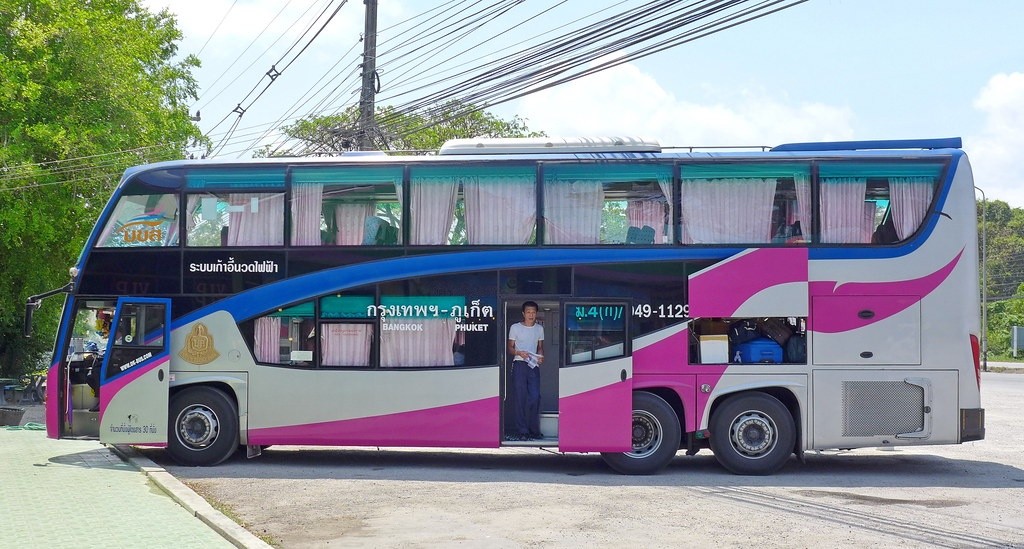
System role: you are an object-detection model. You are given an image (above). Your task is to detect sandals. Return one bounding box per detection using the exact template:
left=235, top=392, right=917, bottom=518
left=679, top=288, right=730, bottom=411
left=530, top=432, right=544, bottom=438
left=518, top=433, right=528, bottom=440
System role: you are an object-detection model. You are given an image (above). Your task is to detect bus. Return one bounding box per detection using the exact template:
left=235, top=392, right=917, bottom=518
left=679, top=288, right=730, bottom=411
left=20, top=134, right=986, bottom=475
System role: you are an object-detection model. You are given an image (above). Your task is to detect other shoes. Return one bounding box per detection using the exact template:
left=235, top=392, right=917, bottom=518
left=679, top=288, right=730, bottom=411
left=90, top=396, right=100, bottom=411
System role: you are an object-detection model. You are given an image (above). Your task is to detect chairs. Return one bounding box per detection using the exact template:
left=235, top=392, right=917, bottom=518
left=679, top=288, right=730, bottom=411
left=360, top=217, right=399, bottom=245
left=625, top=225, right=655, bottom=244
left=321, top=230, right=335, bottom=245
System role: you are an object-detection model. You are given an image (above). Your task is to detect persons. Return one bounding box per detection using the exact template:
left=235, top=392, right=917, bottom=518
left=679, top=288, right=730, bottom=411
left=87, top=322, right=122, bottom=412
left=508, top=301, right=544, bottom=441
left=294, top=336, right=316, bottom=367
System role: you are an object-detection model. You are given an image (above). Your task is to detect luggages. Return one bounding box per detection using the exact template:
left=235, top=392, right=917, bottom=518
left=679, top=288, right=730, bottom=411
left=733, top=338, right=783, bottom=363
left=758, top=317, right=792, bottom=346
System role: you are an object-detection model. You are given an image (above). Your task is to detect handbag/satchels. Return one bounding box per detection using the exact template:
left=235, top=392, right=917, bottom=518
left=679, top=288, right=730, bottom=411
left=786, top=336, right=804, bottom=362
left=727, top=320, right=760, bottom=343
